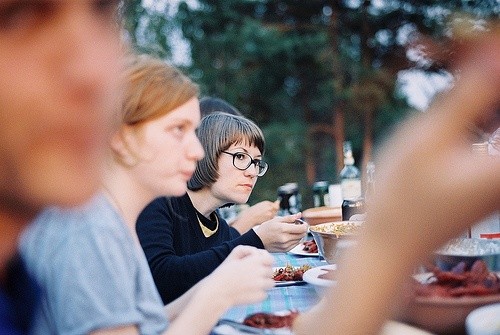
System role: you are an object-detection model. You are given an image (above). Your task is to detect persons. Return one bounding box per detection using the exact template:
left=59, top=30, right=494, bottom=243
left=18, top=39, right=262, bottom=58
left=136, top=112, right=308, bottom=305
left=0, top=0, right=499, bottom=335
left=16, top=54, right=275, bottom=335
left=200, top=95, right=280, bottom=240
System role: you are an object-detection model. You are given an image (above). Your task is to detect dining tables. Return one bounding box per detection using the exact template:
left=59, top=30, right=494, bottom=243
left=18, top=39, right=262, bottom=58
left=219, top=216, right=326, bottom=335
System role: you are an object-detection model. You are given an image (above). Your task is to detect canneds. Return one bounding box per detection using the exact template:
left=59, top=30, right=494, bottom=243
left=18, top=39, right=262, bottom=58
left=312, top=181, right=330, bottom=207
left=278, top=182, right=302, bottom=216
left=341, top=197, right=366, bottom=221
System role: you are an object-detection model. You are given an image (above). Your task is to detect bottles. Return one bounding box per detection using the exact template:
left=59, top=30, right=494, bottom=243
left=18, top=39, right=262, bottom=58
left=365, top=160, right=379, bottom=207
left=340, top=140, right=362, bottom=202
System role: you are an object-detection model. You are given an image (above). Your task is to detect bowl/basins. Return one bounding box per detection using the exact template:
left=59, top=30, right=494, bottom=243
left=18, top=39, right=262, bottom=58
left=405, top=296, right=500, bottom=330
left=309, top=220, right=364, bottom=264
left=301, top=264, right=337, bottom=301
left=432, top=237, right=499, bottom=275
left=301, top=205, right=341, bottom=226
left=465, top=303, right=500, bottom=335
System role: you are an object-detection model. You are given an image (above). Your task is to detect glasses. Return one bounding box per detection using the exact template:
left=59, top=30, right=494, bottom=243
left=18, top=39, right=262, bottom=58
left=221, top=150, right=269, bottom=177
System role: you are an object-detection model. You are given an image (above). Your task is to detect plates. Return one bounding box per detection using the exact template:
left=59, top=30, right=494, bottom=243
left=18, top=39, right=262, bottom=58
left=288, top=243, right=320, bottom=257
left=273, top=266, right=310, bottom=286
